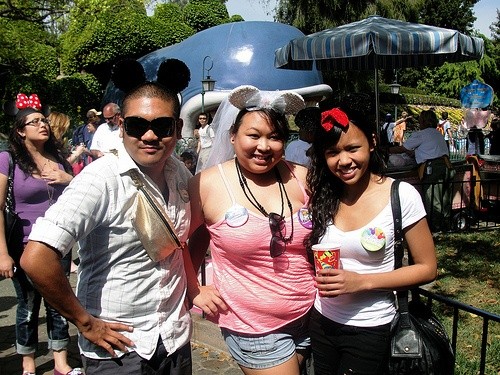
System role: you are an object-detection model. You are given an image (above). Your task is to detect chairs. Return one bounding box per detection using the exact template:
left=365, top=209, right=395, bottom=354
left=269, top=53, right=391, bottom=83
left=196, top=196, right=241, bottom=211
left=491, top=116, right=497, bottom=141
left=421, top=158, right=447, bottom=221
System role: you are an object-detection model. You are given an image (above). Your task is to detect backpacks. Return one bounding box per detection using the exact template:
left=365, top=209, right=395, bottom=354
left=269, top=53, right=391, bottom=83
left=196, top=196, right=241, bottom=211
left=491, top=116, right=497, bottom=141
left=380, top=123, right=390, bottom=144
left=437, top=120, right=447, bottom=136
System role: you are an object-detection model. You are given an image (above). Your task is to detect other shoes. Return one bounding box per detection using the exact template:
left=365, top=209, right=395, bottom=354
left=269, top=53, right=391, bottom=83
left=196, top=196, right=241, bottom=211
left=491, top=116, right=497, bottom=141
left=54, top=366, right=84, bottom=375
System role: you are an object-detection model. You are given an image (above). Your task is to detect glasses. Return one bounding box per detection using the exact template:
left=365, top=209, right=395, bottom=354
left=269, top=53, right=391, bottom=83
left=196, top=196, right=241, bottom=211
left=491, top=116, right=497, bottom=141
left=269, top=213, right=286, bottom=258
left=200, top=117, right=207, bottom=121
left=122, top=115, right=175, bottom=139
left=105, top=112, right=117, bottom=121
left=24, top=118, right=51, bottom=127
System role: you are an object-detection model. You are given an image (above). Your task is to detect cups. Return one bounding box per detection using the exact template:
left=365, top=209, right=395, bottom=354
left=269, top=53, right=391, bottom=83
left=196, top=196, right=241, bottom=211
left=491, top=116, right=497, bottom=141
left=311, top=242, right=342, bottom=298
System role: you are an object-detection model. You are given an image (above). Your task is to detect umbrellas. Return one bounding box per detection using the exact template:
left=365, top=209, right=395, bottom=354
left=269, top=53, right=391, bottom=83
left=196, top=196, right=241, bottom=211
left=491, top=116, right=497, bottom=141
left=274, top=15, right=484, bottom=137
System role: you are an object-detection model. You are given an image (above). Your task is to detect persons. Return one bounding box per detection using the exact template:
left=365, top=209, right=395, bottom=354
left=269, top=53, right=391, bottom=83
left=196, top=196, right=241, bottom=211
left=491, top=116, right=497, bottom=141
left=179, top=113, right=214, bottom=175
left=294, top=107, right=437, bottom=375
left=21, top=57, right=209, bottom=375
left=0, top=93, right=84, bottom=375
left=285, top=129, right=313, bottom=168
left=85, top=108, right=101, bottom=166
left=184, top=85, right=317, bottom=375
left=381, top=112, right=413, bottom=146
left=439, top=112, right=500, bottom=155
left=90, top=103, right=125, bottom=160
left=387, top=111, right=450, bottom=196
left=48, top=111, right=84, bottom=165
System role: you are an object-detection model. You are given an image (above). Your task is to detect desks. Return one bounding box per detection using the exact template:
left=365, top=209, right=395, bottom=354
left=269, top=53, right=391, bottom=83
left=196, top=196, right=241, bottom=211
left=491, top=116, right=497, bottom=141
left=384, top=164, right=474, bottom=215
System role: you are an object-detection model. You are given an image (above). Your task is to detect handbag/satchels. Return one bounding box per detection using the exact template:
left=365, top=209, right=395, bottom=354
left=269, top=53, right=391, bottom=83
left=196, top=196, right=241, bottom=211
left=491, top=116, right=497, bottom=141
left=0, top=149, right=20, bottom=281
left=128, top=170, right=183, bottom=261
left=390, top=300, right=455, bottom=375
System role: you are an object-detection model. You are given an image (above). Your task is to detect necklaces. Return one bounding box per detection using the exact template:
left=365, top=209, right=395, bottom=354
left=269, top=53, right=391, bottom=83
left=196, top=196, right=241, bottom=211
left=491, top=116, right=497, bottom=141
left=33, top=156, right=55, bottom=206
left=234, top=157, right=293, bottom=243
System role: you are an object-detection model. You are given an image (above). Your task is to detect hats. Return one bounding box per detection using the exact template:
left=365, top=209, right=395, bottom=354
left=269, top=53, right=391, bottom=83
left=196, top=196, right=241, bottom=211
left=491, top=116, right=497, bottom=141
left=87, top=109, right=102, bottom=118
